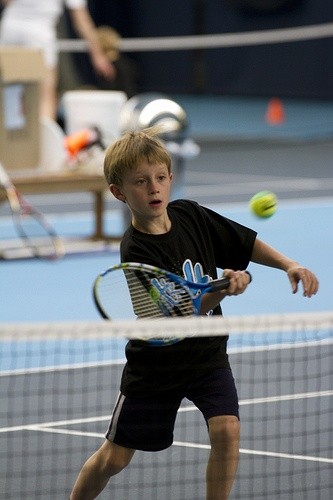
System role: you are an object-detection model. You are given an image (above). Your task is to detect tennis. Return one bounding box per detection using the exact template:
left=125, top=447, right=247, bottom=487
left=251, top=192, right=278, bottom=219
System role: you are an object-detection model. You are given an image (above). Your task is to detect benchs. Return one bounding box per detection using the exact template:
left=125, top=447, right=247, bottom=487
left=0, top=166, right=137, bottom=260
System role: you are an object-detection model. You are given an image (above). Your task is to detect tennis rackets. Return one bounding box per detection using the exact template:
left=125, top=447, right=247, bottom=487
left=92, top=261, right=251, bottom=347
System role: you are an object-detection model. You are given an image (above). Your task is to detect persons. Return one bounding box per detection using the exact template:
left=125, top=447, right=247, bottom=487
left=1, top=0, right=114, bottom=122
left=68, top=128, right=322, bottom=500
